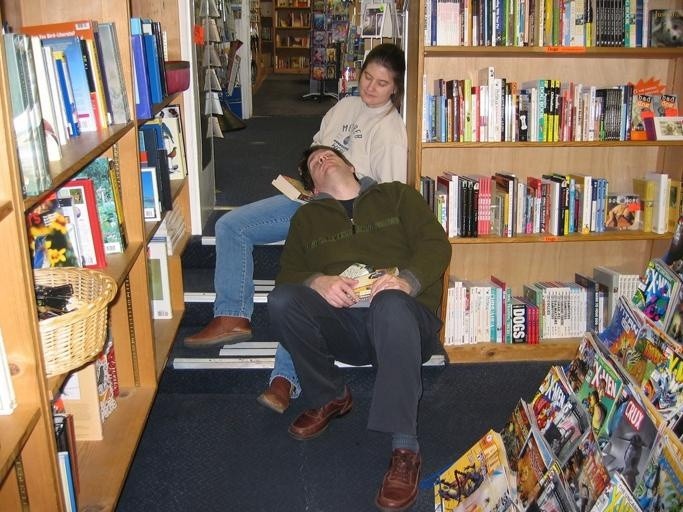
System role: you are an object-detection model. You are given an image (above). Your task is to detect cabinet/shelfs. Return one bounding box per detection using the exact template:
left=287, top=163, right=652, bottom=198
left=180, top=1, right=263, bottom=129
left=272, top=2, right=308, bottom=76
left=303, top=1, right=404, bottom=102
left=405, top=0, right=683, bottom=365
left=0, top=0, right=191, bottom=512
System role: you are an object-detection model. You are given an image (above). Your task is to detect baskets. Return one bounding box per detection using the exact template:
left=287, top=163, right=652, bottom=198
left=30, top=265, right=117, bottom=377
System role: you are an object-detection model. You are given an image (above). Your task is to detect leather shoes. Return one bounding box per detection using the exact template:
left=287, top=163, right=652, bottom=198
left=257, top=377, right=290, bottom=414
left=374, top=445, right=422, bottom=510
left=288, top=384, right=353, bottom=441
left=183, top=315, right=254, bottom=350
